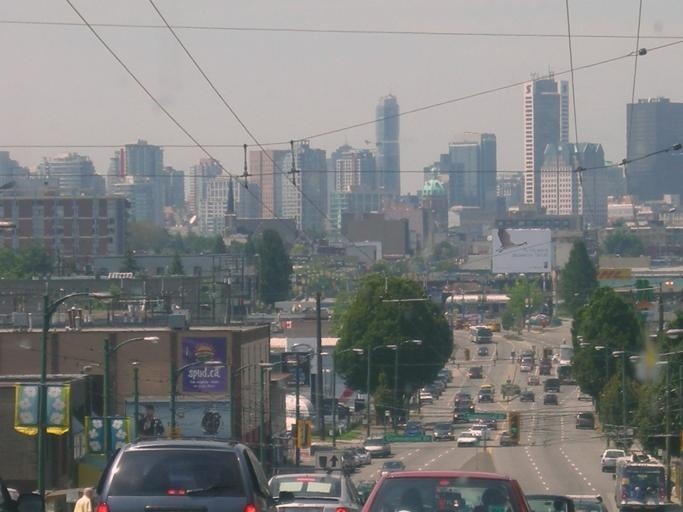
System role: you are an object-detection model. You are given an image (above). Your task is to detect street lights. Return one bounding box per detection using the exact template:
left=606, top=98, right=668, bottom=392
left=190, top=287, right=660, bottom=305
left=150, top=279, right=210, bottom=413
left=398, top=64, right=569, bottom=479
left=658, top=280, right=673, bottom=333
left=519, top=272, right=532, bottom=332
left=168, top=357, right=220, bottom=438
left=227, top=336, right=421, bottom=477
left=102, top=333, right=162, bottom=456
left=576, top=327, right=683, bottom=503
left=36, top=284, right=118, bottom=495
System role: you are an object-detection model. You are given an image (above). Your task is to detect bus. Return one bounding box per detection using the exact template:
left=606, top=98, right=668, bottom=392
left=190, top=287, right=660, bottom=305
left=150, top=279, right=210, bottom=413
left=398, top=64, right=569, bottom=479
left=614, top=452, right=665, bottom=510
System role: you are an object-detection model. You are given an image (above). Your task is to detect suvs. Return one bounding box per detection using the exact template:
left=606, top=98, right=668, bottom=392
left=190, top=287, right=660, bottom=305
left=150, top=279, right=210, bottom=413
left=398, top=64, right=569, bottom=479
left=94, top=437, right=278, bottom=512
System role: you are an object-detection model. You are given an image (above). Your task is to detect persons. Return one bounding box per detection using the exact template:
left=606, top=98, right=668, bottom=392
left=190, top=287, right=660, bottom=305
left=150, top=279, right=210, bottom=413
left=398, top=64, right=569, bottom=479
left=138, top=405, right=165, bottom=439
left=73, top=487, right=95, bottom=512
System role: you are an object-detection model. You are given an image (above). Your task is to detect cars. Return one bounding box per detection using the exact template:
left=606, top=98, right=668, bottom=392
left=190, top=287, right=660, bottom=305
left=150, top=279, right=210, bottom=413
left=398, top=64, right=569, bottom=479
left=363, top=469, right=531, bottom=511
left=523, top=493, right=573, bottom=511
left=420, top=314, right=574, bottom=446
left=564, top=492, right=608, bottom=511
left=266, top=472, right=363, bottom=512
